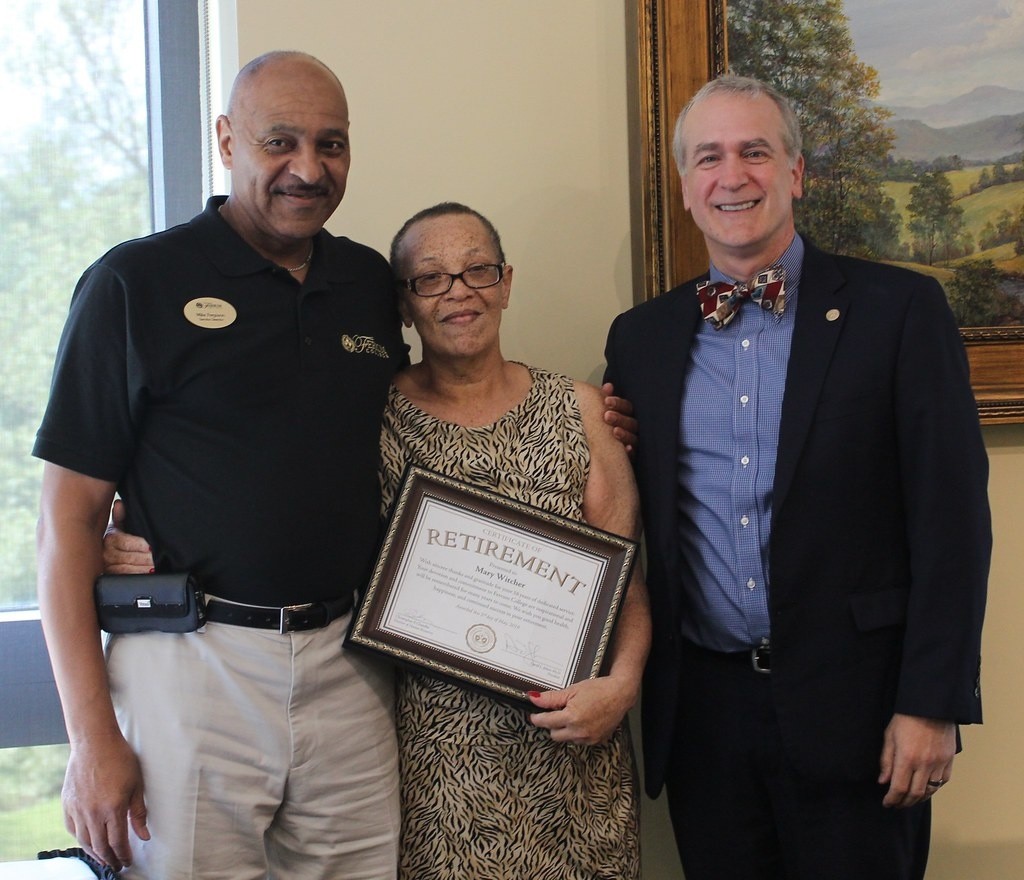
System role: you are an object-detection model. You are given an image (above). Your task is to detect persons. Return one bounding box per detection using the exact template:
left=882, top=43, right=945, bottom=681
left=31, top=49, right=638, bottom=880
left=600, top=75, right=992, bottom=880
left=100, top=203, right=654, bottom=880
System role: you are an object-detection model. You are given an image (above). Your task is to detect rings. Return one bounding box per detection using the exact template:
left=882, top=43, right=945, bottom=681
left=927, top=779, right=944, bottom=788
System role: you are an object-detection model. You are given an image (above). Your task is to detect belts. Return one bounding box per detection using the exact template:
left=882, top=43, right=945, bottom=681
left=205, top=589, right=366, bottom=633
left=679, top=634, right=772, bottom=680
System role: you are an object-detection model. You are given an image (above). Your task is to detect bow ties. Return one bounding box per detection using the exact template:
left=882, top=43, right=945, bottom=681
left=696, top=265, right=787, bottom=331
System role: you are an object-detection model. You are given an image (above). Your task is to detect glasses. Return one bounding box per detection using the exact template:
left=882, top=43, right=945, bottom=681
left=402, top=261, right=504, bottom=296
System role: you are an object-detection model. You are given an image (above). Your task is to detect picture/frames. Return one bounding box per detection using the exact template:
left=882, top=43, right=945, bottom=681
left=342, top=462, right=641, bottom=714
left=637, top=2, right=1024, bottom=426
